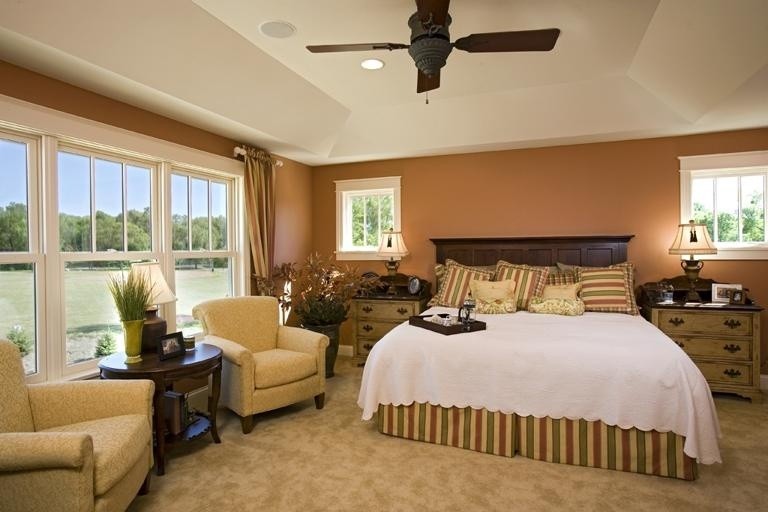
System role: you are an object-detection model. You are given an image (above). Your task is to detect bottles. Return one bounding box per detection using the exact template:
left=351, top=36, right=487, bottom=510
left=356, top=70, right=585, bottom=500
left=140, top=307, right=168, bottom=355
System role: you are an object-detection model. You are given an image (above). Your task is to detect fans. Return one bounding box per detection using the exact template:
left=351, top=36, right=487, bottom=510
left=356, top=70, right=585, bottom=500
left=299, top=1, right=566, bottom=108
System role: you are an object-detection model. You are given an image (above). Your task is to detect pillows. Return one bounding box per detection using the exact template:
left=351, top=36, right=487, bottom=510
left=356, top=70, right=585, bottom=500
left=421, top=256, right=642, bottom=319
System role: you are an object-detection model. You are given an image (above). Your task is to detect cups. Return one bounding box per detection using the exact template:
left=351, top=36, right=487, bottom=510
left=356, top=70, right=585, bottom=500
left=430, top=312, right=458, bottom=327
left=458, top=298, right=476, bottom=325
left=656, top=280, right=674, bottom=305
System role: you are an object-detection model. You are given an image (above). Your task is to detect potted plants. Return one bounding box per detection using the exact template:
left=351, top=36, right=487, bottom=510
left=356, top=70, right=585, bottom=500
left=104, top=269, right=165, bottom=365
left=250, top=248, right=362, bottom=378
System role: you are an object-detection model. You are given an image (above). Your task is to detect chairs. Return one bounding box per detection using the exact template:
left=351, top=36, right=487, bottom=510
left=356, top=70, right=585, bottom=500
left=2, top=333, right=166, bottom=512
left=190, top=293, right=331, bottom=437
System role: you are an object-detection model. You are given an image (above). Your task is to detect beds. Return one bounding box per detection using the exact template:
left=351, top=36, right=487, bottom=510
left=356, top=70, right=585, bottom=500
left=354, top=232, right=728, bottom=485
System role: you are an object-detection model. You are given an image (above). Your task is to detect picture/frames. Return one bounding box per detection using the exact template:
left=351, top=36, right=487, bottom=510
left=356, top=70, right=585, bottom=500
left=728, top=289, right=747, bottom=306
left=710, top=282, right=742, bottom=304
left=154, top=330, right=188, bottom=362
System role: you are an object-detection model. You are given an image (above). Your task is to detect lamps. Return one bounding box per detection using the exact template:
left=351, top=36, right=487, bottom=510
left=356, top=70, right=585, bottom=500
left=666, top=218, right=719, bottom=304
left=378, top=232, right=410, bottom=296
left=126, top=261, right=184, bottom=354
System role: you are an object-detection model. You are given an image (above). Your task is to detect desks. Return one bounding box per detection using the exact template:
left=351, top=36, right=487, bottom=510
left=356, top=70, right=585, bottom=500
left=95, top=337, right=228, bottom=478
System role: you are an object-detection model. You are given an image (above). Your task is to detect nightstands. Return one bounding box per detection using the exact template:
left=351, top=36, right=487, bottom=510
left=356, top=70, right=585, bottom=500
left=639, top=276, right=766, bottom=406
left=350, top=270, right=432, bottom=370
